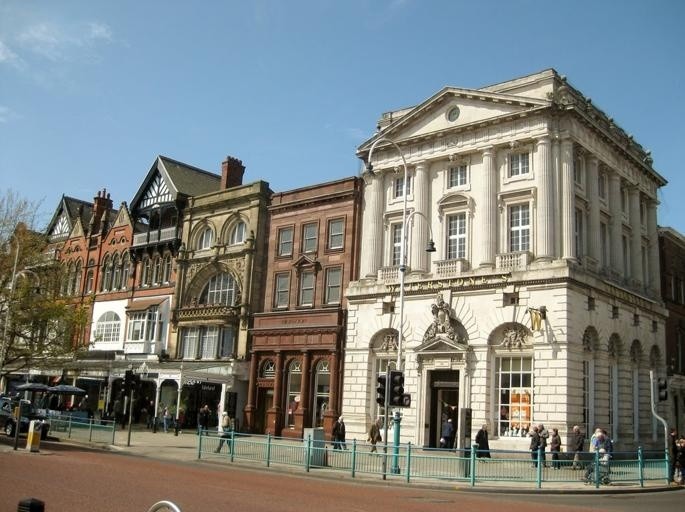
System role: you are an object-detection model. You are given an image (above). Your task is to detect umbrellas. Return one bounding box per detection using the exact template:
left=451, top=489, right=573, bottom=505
left=15, top=382, right=48, bottom=401
left=43, top=383, right=86, bottom=408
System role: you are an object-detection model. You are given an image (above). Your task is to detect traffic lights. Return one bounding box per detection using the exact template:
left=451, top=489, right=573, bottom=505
left=388, top=371, right=404, bottom=407
left=376, top=375, right=386, bottom=407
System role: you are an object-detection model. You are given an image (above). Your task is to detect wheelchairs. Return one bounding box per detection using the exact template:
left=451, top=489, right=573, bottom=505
left=580, top=453, right=613, bottom=485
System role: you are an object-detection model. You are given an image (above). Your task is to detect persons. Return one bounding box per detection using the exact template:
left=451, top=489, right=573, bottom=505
left=340, top=415, right=349, bottom=450
left=440, top=418, right=455, bottom=448
left=200, top=404, right=212, bottom=436
left=669, top=427, right=679, bottom=485
left=672, top=439, right=680, bottom=481
left=332, top=417, right=343, bottom=452
left=112, top=392, right=183, bottom=436
left=368, top=419, right=383, bottom=457
left=78, top=394, right=93, bottom=418
left=214, top=411, right=235, bottom=454
left=528, top=422, right=614, bottom=483
left=678, top=438, right=685, bottom=485
left=476, top=423, right=491, bottom=463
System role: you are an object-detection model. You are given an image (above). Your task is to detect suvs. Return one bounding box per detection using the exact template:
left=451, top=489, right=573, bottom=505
left=0, top=397, right=51, bottom=437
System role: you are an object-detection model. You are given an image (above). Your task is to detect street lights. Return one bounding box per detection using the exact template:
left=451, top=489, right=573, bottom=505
left=366, top=138, right=435, bottom=475
left=0, top=232, right=40, bottom=371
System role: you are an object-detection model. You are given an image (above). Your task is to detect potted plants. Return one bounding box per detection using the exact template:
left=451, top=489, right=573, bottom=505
left=169, top=396, right=189, bottom=425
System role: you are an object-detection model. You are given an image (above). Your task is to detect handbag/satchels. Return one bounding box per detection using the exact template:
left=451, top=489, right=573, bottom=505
left=440, top=437, right=445, bottom=443
left=224, top=426, right=231, bottom=432
